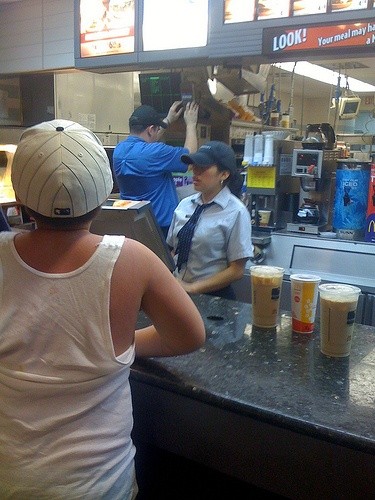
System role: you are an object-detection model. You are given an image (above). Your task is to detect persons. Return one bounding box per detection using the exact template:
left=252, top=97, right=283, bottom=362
left=166, top=141, right=253, bottom=301
left=0, top=118, right=206, bottom=500
left=113, top=100, right=199, bottom=252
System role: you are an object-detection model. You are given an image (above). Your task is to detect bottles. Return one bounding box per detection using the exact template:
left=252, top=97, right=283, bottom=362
left=282, top=113, right=290, bottom=128
left=270, top=109, right=279, bottom=127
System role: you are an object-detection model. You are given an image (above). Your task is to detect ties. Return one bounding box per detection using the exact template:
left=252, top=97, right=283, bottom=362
left=175, top=200, right=216, bottom=269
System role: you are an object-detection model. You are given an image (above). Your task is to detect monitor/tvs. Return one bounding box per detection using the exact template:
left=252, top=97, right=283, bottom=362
left=94, top=199, right=175, bottom=272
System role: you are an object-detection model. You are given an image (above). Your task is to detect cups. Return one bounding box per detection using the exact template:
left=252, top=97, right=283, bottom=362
left=318, top=283, right=362, bottom=358
left=289, top=274, right=321, bottom=333
left=259, top=210, right=271, bottom=226
left=250, top=265, right=286, bottom=328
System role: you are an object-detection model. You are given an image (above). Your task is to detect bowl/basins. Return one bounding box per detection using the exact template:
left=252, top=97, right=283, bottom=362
left=261, top=130, right=291, bottom=139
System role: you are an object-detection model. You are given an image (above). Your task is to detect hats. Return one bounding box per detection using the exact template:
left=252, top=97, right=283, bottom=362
left=181, top=138, right=236, bottom=176
left=128, top=105, right=167, bottom=129
left=11, top=118, right=114, bottom=217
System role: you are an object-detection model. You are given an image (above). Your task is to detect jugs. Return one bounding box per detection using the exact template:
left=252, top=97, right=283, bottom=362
left=301, top=123, right=338, bottom=150
left=296, top=200, right=322, bottom=225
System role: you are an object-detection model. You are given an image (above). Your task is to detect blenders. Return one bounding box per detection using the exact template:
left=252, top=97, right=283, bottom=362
left=286, top=148, right=341, bottom=235
left=244, top=138, right=303, bottom=232
